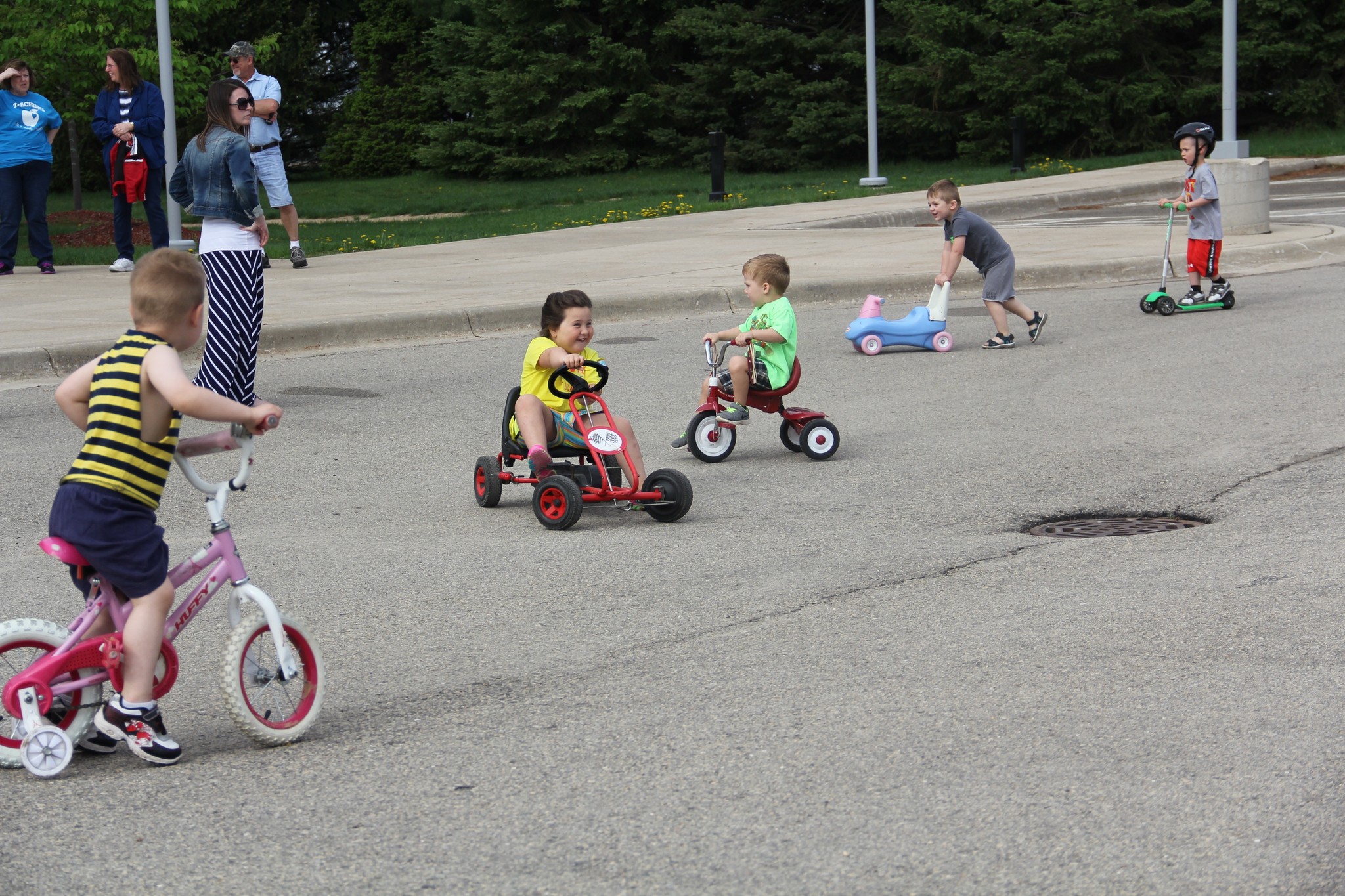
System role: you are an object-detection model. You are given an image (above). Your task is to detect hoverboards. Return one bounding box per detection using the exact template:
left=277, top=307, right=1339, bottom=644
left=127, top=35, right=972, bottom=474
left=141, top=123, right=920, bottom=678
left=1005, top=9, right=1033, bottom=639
left=1139, top=201, right=1235, bottom=316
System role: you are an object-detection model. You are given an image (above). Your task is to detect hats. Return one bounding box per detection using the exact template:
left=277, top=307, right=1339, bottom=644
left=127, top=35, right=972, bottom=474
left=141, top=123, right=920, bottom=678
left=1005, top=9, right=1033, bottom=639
left=221, top=41, right=256, bottom=59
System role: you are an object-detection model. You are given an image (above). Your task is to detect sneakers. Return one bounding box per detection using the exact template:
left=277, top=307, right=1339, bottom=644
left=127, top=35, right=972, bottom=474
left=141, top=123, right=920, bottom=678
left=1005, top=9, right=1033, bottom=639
left=40, top=697, right=118, bottom=754
left=290, top=246, right=308, bottom=269
left=715, top=402, right=751, bottom=425
left=41, top=262, right=55, bottom=274
left=630, top=491, right=646, bottom=511
left=1178, top=288, right=1205, bottom=306
left=93, top=691, right=183, bottom=764
left=109, top=257, right=134, bottom=272
left=1206, top=278, right=1231, bottom=303
left=671, top=427, right=689, bottom=450
left=527, top=445, right=557, bottom=484
left=261, top=252, right=271, bottom=268
left=0, top=262, right=14, bottom=275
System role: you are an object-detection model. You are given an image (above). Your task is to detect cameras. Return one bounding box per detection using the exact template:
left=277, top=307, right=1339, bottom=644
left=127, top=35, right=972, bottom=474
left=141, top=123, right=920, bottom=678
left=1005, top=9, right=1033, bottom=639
left=265, top=119, right=272, bottom=125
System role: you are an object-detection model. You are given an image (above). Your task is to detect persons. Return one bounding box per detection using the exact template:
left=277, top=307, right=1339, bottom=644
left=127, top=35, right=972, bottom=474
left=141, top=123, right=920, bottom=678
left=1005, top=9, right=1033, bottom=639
left=509, top=289, right=651, bottom=511
left=1158, top=122, right=1230, bottom=306
left=47, top=248, right=283, bottom=767
left=926, top=179, right=1048, bottom=350
left=668, top=254, right=798, bottom=451
left=219, top=42, right=307, bottom=268
left=0, top=59, right=62, bottom=276
left=91, top=49, right=171, bottom=274
left=168, top=79, right=270, bottom=415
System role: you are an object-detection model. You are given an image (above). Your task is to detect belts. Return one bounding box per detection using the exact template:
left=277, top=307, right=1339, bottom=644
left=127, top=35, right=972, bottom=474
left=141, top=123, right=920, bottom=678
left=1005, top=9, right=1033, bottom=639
left=250, top=141, right=279, bottom=153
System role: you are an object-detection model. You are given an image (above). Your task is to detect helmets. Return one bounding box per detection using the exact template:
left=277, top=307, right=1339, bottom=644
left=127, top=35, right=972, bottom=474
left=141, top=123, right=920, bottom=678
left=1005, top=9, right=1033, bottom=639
left=1171, top=122, right=1215, bottom=157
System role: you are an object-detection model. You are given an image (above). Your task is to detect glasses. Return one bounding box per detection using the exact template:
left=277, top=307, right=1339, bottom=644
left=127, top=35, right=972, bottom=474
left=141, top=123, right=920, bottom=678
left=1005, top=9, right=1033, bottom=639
left=229, top=96, right=255, bottom=110
left=228, top=57, right=245, bottom=64
left=106, top=64, right=117, bottom=68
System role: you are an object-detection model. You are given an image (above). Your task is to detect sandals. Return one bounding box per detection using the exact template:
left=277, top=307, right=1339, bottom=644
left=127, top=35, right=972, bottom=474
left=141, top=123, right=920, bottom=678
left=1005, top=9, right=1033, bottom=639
left=982, top=333, right=1015, bottom=349
left=1027, top=311, right=1048, bottom=343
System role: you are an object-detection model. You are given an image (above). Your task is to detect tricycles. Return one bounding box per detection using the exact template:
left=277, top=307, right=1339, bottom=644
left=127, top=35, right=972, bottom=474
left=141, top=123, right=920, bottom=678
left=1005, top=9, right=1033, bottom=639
left=685, top=337, right=841, bottom=463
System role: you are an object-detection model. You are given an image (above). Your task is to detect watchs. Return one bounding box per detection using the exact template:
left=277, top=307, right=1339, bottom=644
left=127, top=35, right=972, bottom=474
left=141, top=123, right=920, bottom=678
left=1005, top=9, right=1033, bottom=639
left=130, top=121, right=134, bottom=130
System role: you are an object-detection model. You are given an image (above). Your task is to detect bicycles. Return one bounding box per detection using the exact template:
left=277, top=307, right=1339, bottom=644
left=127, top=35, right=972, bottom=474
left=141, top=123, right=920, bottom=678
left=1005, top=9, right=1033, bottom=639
left=0, top=411, right=328, bottom=780
